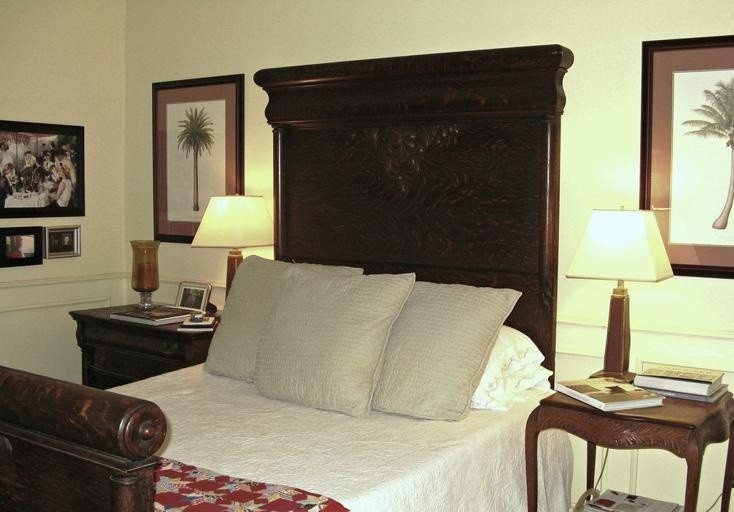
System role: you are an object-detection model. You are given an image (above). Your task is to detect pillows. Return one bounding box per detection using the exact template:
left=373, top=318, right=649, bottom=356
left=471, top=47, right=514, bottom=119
left=373, top=279, right=524, bottom=423
left=253, top=270, right=416, bottom=416
left=203, top=255, right=362, bottom=380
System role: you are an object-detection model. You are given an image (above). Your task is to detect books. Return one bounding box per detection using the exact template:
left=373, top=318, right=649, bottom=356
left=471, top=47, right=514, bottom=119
left=108, top=305, right=219, bottom=333
left=555, top=360, right=730, bottom=412
left=582, top=489, right=684, bottom=512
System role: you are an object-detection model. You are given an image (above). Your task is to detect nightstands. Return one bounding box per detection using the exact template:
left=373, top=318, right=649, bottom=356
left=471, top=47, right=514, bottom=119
left=523, top=375, right=734, bottom=512
left=70, top=302, right=219, bottom=390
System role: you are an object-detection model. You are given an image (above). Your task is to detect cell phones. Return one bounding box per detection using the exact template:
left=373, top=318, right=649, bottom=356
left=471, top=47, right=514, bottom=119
left=191, top=312, right=203, bottom=322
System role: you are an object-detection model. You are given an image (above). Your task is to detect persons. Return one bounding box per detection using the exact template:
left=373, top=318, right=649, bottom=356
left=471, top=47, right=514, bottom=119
left=0, top=140, right=77, bottom=211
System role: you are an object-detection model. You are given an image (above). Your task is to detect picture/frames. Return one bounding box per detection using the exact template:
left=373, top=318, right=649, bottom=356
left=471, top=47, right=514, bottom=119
left=175, top=282, right=212, bottom=311
left=0, top=226, right=45, bottom=268
left=44, top=226, right=80, bottom=259
left=640, top=35, right=734, bottom=279
left=153, top=73, right=244, bottom=244
left=0, top=121, right=84, bottom=218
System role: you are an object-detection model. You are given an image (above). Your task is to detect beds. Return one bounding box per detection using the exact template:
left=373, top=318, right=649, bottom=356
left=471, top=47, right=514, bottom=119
left=2, top=42, right=572, bottom=510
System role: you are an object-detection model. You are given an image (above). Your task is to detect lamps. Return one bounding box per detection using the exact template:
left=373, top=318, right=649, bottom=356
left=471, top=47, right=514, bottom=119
left=191, top=195, right=274, bottom=298
left=130, top=239, right=160, bottom=309
left=566, top=209, right=674, bottom=383
